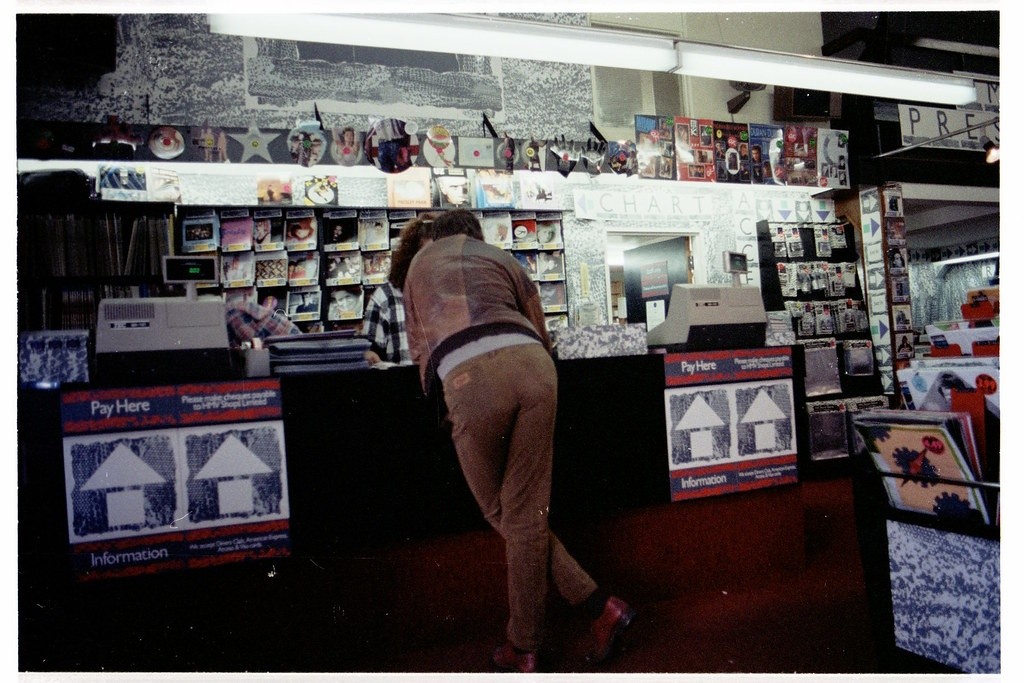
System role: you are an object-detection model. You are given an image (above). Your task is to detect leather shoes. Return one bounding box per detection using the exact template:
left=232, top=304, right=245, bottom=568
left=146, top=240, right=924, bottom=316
left=584, top=592, right=639, bottom=665
left=490, top=641, right=539, bottom=673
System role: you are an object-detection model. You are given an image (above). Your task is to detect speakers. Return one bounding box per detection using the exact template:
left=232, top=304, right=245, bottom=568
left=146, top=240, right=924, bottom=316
left=773, top=86, right=832, bottom=122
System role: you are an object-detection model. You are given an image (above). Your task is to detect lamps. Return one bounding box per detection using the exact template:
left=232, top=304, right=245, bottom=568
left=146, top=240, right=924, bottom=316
left=205, top=14, right=679, bottom=73
left=668, top=41, right=976, bottom=106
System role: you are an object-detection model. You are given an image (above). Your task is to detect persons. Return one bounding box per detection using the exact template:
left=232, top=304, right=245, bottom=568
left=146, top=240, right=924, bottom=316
left=837, top=156, right=846, bottom=170
left=728, top=152, right=738, bottom=170
left=626, top=148, right=637, bottom=169
left=750, top=145, right=761, bottom=164
left=716, top=141, right=725, bottom=159
left=898, top=335, right=912, bottom=352
left=739, top=143, right=748, bottom=160
left=830, top=165, right=839, bottom=179
left=332, top=124, right=361, bottom=167
left=223, top=302, right=303, bottom=344
left=821, top=164, right=830, bottom=178
left=251, top=224, right=362, bottom=319
left=895, top=281, right=904, bottom=297
left=496, top=219, right=563, bottom=274
left=402, top=208, right=634, bottom=673
left=358, top=213, right=439, bottom=366
left=892, top=253, right=903, bottom=269
left=438, top=176, right=470, bottom=208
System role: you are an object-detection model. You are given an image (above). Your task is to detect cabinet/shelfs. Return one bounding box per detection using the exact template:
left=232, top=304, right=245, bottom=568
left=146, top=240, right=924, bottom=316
left=17, top=119, right=192, bottom=331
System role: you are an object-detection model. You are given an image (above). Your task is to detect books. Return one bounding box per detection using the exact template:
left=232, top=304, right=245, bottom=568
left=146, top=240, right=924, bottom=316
left=851, top=286, right=999, bottom=524
left=18, top=215, right=169, bottom=331
left=882, top=189, right=915, bottom=370
left=633, top=114, right=851, bottom=190
